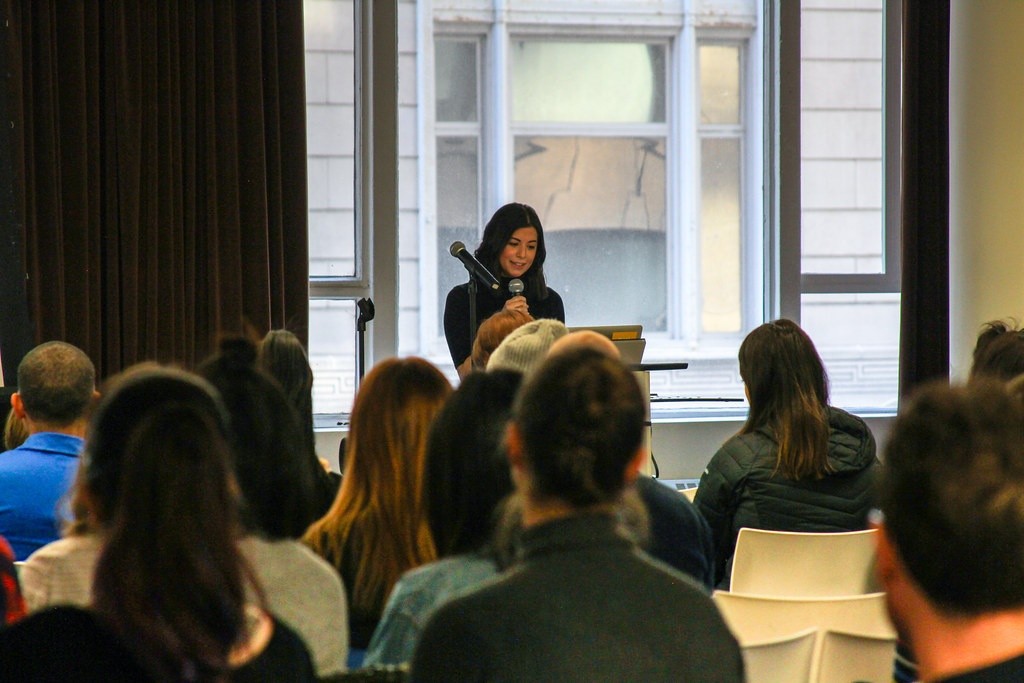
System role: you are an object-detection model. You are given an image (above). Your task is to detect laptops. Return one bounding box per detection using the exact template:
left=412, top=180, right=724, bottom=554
left=564, top=325, right=643, bottom=340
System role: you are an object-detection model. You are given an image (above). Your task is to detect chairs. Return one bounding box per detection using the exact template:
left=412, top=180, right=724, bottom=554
left=711, top=526, right=899, bottom=683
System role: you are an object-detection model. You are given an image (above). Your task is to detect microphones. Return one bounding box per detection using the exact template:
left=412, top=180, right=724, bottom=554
left=509, top=278, right=524, bottom=298
left=449, top=241, right=500, bottom=288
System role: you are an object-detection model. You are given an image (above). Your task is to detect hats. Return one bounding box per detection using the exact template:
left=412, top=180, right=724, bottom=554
left=485, top=318, right=570, bottom=377
left=548, top=329, right=622, bottom=362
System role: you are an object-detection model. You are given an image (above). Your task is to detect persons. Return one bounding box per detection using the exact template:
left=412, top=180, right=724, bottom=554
left=0, top=602, right=178, bottom=683
left=486, top=319, right=717, bottom=593
left=5, top=406, right=29, bottom=450
left=411, top=344, right=746, bottom=683
left=302, top=358, right=456, bottom=651
left=261, top=327, right=342, bottom=519
left=444, top=203, right=565, bottom=382
left=363, top=368, right=527, bottom=682
left=194, top=335, right=347, bottom=677
left=24, top=364, right=349, bottom=683
left=967, top=318, right=1024, bottom=381
left=877, top=382, right=1024, bottom=683
left=693, top=319, right=884, bottom=591
left=472, top=309, right=535, bottom=372
left=0, top=343, right=94, bottom=560
